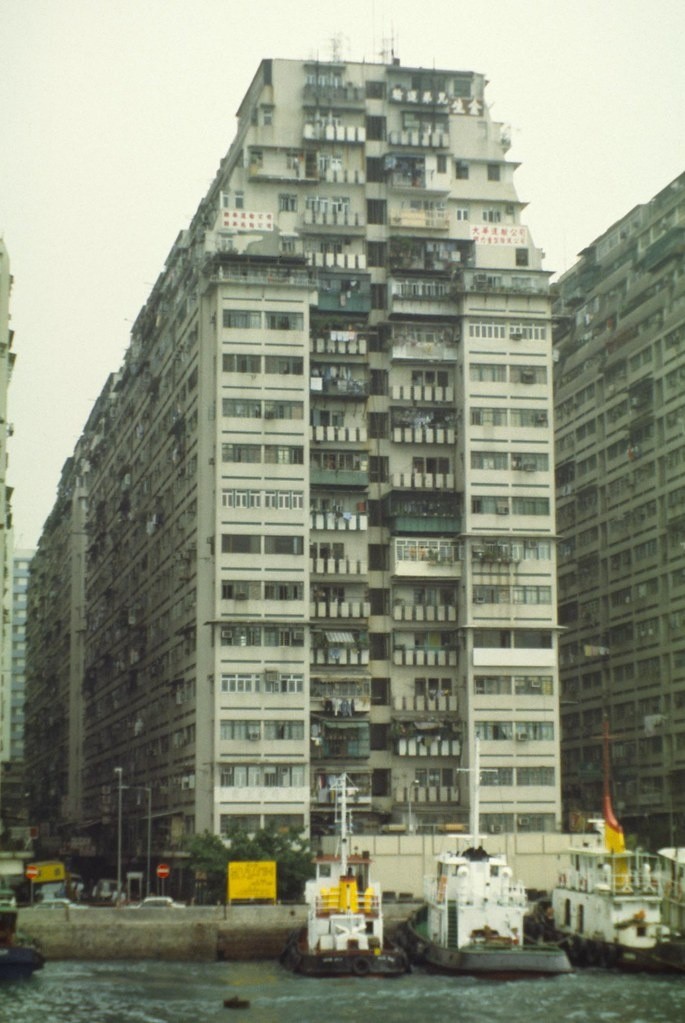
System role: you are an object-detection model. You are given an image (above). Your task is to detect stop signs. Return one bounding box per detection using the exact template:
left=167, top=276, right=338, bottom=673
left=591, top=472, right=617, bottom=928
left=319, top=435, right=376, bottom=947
left=25, top=866, right=39, bottom=880
left=157, top=864, right=170, bottom=878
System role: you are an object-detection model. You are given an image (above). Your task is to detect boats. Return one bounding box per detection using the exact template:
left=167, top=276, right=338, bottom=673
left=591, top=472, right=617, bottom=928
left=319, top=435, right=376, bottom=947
left=534, top=712, right=685, bottom=973
left=405, top=737, right=575, bottom=973
left=0, top=889, right=45, bottom=979
left=278, top=772, right=410, bottom=976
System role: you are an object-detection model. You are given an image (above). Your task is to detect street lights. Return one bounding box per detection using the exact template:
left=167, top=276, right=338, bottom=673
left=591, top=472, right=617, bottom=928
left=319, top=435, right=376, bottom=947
left=114, top=767, right=152, bottom=896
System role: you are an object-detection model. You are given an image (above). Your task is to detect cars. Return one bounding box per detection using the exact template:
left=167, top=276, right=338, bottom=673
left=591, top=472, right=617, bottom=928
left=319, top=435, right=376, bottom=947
left=32, top=898, right=89, bottom=911
left=123, top=896, right=186, bottom=909
